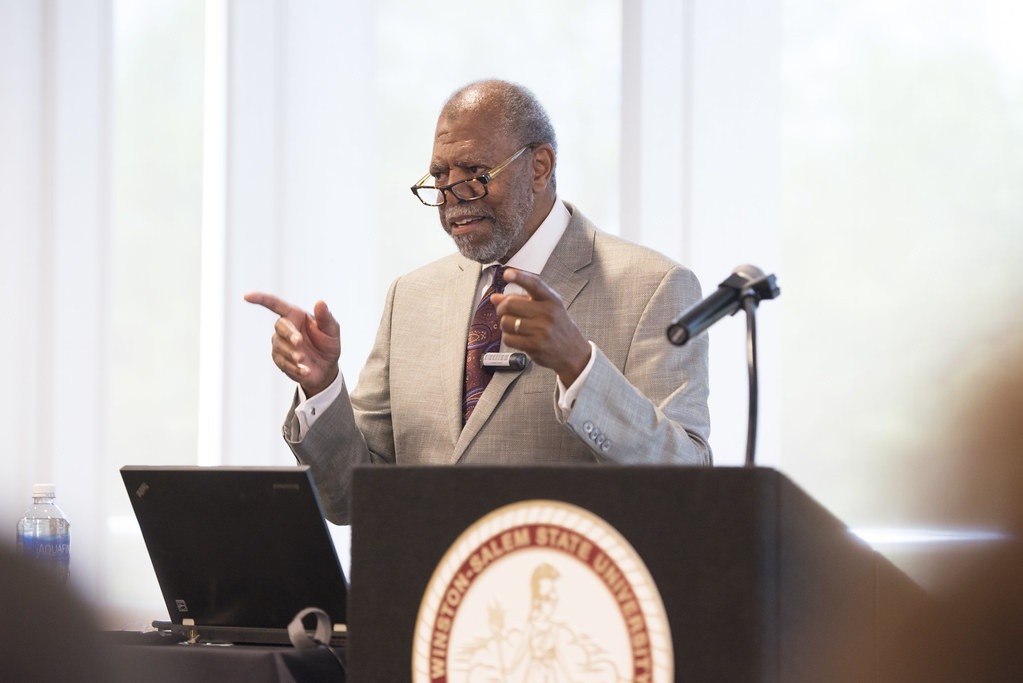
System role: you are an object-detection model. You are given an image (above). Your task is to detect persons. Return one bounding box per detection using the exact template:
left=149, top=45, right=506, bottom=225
left=245, top=80, right=712, bottom=525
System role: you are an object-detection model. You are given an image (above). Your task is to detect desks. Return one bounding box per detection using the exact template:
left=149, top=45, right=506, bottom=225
left=109, top=645, right=346, bottom=683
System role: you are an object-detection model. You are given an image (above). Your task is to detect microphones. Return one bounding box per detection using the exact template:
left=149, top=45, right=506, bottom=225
left=667, top=265, right=764, bottom=347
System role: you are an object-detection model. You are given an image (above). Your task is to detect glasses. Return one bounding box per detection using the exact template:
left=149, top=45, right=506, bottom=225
left=410, top=142, right=543, bottom=207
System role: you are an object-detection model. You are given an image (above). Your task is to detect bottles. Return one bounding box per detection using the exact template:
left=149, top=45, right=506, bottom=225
left=16, top=483, right=71, bottom=595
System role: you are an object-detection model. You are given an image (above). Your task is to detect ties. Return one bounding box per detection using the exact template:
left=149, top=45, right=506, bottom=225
left=461, top=265, right=513, bottom=429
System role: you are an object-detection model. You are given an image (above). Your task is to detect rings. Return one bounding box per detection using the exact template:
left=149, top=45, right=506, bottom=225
left=514, top=318, right=521, bottom=333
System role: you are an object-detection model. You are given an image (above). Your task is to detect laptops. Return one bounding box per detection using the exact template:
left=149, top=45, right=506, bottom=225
left=121, top=465, right=349, bottom=647
